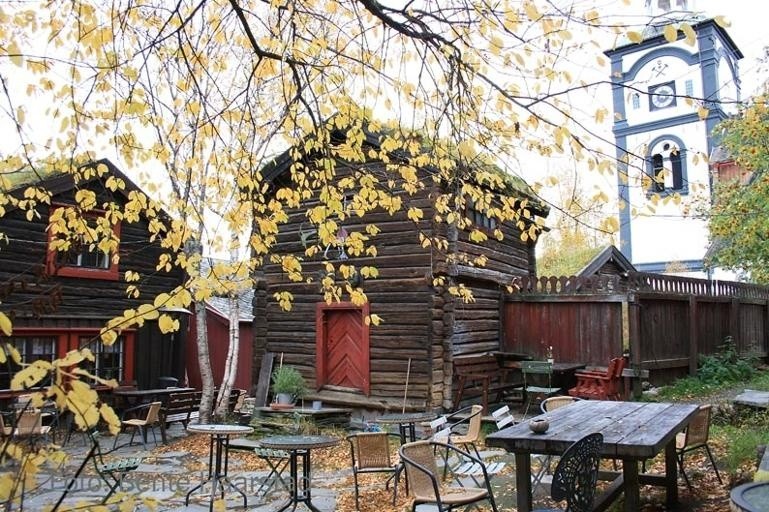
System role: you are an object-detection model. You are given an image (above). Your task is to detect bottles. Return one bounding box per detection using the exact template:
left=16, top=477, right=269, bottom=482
left=547, top=346, right=555, bottom=365
left=530, top=420, right=549, bottom=433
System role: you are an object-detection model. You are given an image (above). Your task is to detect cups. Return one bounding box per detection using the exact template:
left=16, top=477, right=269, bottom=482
left=313, top=401, right=321, bottom=410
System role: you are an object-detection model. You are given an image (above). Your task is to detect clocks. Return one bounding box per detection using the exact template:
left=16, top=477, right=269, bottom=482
left=651, top=85, right=675, bottom=108
